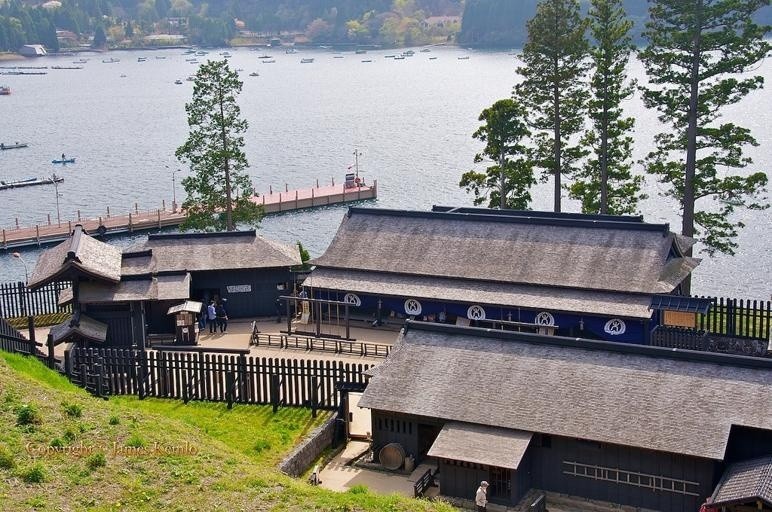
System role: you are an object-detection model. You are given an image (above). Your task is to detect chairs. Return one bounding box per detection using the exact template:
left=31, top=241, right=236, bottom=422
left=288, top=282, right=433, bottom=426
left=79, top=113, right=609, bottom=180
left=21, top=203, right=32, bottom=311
left=220, top=331, right=229, bottom=335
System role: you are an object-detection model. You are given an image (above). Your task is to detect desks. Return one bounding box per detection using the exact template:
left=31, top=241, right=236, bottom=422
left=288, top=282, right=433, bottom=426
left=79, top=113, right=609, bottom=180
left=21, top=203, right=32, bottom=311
left=13, top=252, right=29, bottom=286
left=165, top=165, right=181, bottom=203
left=48, top=177, right=64, bottom=226
left=349, top=148, right=363, bottom=179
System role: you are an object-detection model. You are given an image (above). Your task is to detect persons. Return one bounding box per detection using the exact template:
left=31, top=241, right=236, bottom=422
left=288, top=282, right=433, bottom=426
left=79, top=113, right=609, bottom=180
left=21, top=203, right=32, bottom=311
left=195, top=292, right=232, bottom=337
left=274, top=285, right=325, bottom=323
left=475, top=480, right=489, bottom=512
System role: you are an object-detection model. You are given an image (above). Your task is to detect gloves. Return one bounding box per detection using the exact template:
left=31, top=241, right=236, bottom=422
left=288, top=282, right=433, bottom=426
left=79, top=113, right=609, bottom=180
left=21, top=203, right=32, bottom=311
left=0, top=140, right=77, bottom=189
left=332, top=46, right=517, bottom=63
left=0, top=56, right=127, bottom=78
left=0, top=86, right=11, bottom=95
left=138, top=45, right=315, bottom=85
